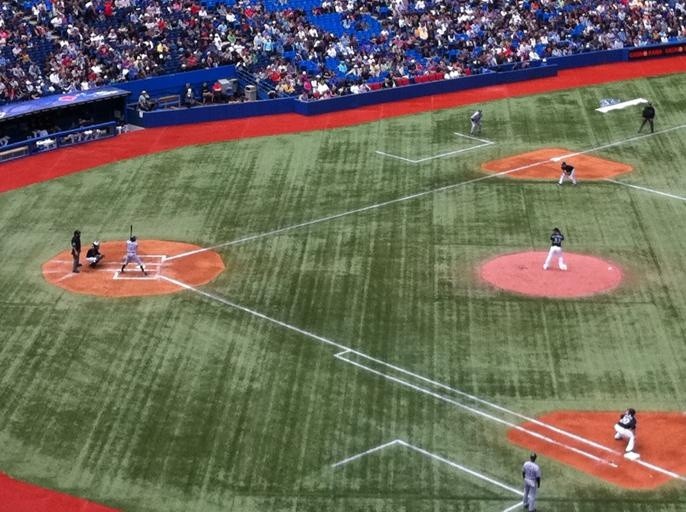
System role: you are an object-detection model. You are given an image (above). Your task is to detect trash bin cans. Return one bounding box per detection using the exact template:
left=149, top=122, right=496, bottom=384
left=244, top=85, right=256, bottom=101
left=231, top=79, right=238, bottom=93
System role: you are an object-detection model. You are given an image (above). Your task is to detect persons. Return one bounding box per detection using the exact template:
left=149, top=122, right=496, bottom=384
left=70, top=230, right=82, bottom=274
left=521, top=452, right=541, bottom=512
left=84, top=241, right=103, bottom=269
left=542, top=228, right=568, bottom=271
left=613, top=408, right=638, bottom=453
left=558, top=161, right=577, bottom=185
left=119, top=235, right=148, bottom=276
left=1, top=0, right=685, bottom=157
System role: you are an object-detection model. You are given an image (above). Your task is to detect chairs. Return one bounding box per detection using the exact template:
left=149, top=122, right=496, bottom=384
left=1, top=1, right=685, bottom=107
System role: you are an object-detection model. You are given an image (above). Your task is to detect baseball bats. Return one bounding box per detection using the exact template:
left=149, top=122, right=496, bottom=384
left=129, top=225, right=133, bottom=240
